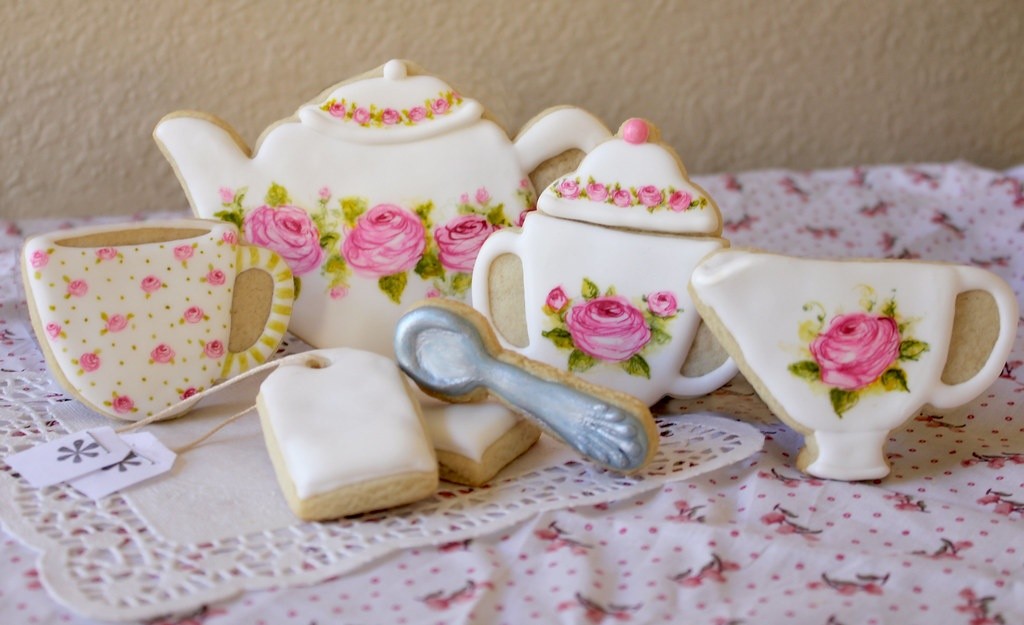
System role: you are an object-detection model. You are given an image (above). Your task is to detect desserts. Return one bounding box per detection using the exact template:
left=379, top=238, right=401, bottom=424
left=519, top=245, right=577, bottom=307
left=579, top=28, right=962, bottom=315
left=21, top=58, right=1017, bottom=522
